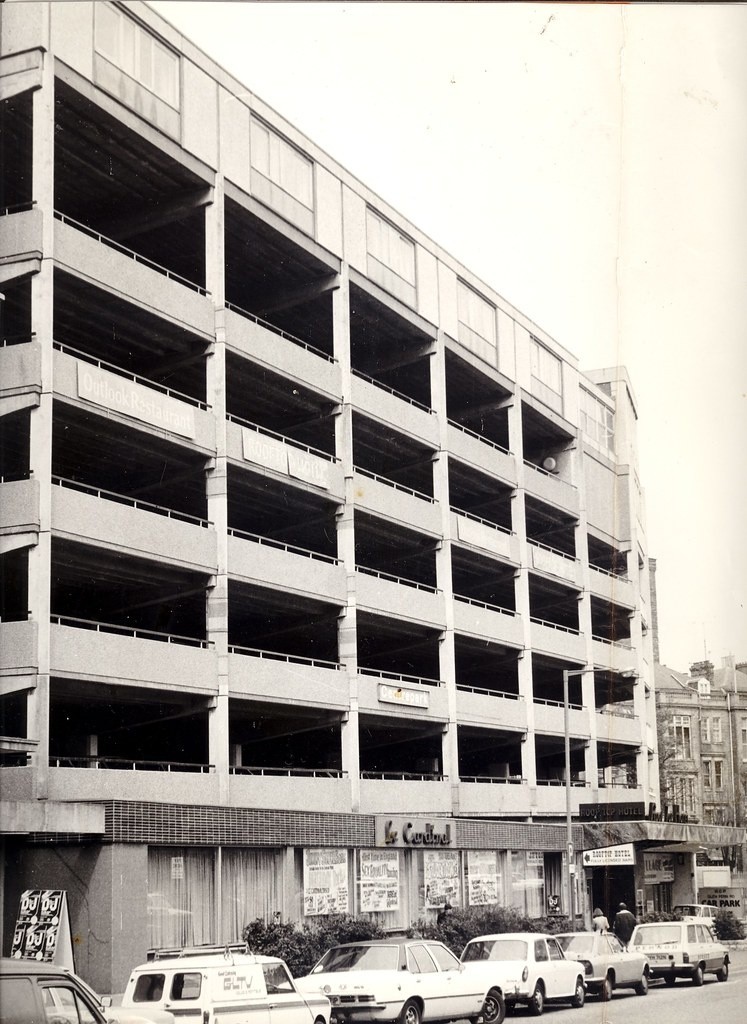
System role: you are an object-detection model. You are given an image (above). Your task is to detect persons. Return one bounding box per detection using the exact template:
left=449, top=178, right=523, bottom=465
left=613, top=903, right=637, bottom=944
left=592, top=908, right=610, bottom=932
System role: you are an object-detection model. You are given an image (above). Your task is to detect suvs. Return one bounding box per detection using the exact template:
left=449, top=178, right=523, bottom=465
left=121, top=941, right=332, bottom=1024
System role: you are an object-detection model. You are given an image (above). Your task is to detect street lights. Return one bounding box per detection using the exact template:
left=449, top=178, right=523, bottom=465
left=563, top=664, right=644, bottom=932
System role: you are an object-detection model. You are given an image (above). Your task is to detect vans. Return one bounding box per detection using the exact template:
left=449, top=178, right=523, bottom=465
left=672, top=904, right=719, bottom=936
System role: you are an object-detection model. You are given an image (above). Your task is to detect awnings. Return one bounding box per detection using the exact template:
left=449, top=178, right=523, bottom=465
left=583, top=821, right=747, bottom=850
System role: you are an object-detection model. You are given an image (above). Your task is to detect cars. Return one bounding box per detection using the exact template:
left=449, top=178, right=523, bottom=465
left=0, top=956, right=176, bottom=1024
left=627, top=921, right=731, bottom=986
left=458, top=932, right=587, bottom=1016
left=277, top=937, right=506, bottom=1024
left=552, top=931, right=654, bottom=1002
